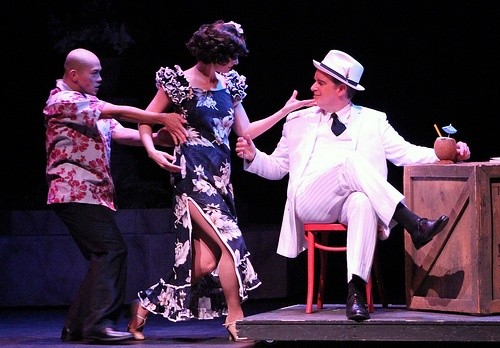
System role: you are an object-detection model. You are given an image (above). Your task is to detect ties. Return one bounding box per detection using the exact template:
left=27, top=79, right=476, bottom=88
left=330, top=112, right=347, bottom=136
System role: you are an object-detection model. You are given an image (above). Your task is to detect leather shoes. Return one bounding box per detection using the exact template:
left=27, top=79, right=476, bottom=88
left=61, top=324, right=134, bottom=344
left=410, top=215, right=449, bottom=250
left=345, top=293, right=370, bottom=319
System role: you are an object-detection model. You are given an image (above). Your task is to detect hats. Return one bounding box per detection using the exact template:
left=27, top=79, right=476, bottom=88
left=312, top=50, right=365, bottom=91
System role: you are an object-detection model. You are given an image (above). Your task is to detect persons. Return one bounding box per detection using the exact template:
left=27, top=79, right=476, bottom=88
left=236, top=50, right=471, bottom=319
left=126, top=21, right=317, bottom=342
left=43, top=47, right=188, bottom=342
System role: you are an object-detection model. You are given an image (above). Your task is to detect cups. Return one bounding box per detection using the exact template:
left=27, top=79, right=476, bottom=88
left=435, top=137, right=458, bottom=163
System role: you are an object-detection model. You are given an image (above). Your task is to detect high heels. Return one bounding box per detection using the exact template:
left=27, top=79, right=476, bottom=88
left=222, top=317, right=249, bottom=342
left=124, top=299, right=147, bottom=340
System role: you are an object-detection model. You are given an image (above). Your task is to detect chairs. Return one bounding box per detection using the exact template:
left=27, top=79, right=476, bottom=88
left=303, top=223, right=373, bottom=313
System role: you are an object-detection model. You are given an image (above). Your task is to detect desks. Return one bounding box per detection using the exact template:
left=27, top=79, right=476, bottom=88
left=403, top=162, right=500, bottom=315
